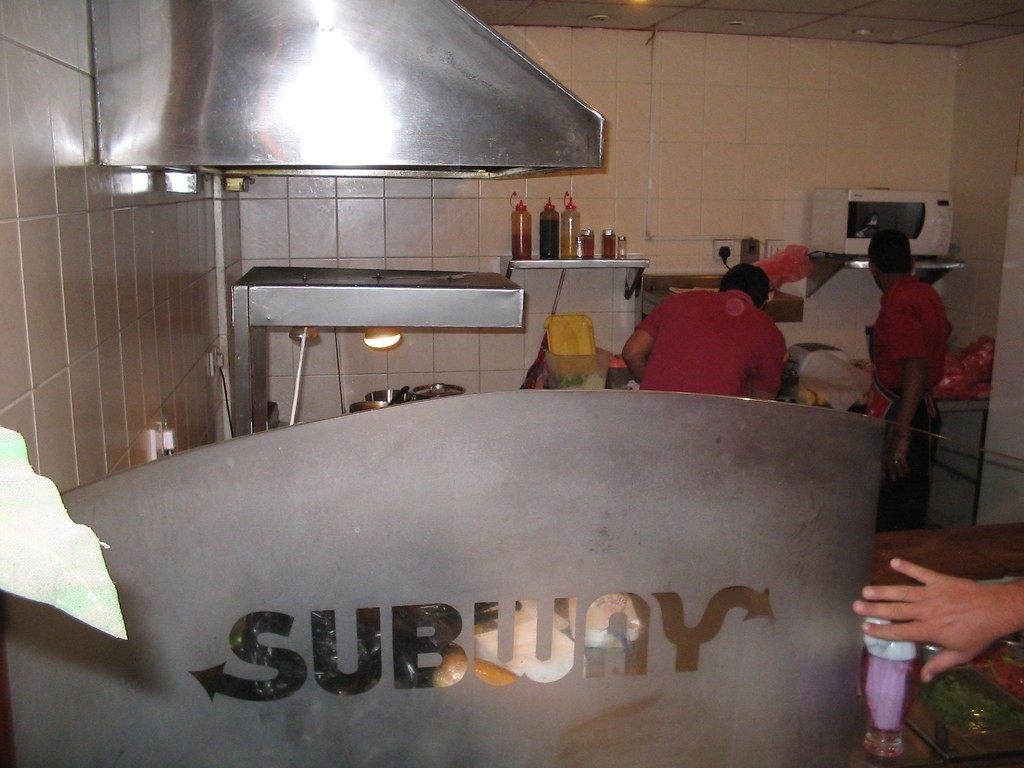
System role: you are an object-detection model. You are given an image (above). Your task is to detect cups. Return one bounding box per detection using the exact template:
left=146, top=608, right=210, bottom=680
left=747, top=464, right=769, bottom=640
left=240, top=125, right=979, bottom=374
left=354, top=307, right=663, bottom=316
left=863, top=638, right=919, bottom=754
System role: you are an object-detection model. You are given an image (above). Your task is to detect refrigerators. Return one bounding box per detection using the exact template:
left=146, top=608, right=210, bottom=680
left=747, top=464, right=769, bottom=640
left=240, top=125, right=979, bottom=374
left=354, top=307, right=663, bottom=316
left=974, top=177, right=1024, bottom=527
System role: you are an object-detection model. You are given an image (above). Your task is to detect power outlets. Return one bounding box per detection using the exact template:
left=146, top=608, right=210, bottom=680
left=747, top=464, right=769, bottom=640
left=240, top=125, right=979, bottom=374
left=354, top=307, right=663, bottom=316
left=151, top=417, right=170, bottom=460
left=209, top=345, right=218, bottom=377
left=714, top=239, right=733, bottom=260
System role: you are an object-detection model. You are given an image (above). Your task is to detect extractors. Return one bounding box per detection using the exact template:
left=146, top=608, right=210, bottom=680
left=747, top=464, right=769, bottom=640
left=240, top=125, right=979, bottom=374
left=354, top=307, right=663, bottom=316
left=89, top=0, right=609, bottom=175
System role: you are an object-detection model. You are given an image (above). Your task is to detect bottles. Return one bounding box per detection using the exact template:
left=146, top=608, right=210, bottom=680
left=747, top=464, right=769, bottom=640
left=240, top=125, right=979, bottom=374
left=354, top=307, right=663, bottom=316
left=581, top=229, right=595, bottom=259
left=618, top=235, right=626, bottom=258
left=602, top=229, right=610, bottom=256
left=576, top=236, right=585, bottom=260
left=540, top=197, right=559, bottom=259
left=510, top=191, right=531, bottom=259
left=562, top=190, right=580, bottom=259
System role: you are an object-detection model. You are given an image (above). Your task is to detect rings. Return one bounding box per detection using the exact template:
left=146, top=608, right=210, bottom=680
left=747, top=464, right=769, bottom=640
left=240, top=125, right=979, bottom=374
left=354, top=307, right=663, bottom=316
left=894, top=460, right=901, bottom=464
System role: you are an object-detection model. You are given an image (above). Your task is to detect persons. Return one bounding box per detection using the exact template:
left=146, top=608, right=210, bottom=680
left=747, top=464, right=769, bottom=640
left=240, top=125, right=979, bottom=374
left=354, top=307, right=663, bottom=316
left=622, top=263, right=786, bottom=401
left=868, top=230, right=950, bottom=533
left=853, top=558, right=1024, bottom=684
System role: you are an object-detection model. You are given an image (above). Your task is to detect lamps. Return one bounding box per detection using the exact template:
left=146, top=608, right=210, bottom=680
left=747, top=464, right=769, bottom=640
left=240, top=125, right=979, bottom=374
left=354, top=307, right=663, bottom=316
left=364, top=327, right=401, bottom=348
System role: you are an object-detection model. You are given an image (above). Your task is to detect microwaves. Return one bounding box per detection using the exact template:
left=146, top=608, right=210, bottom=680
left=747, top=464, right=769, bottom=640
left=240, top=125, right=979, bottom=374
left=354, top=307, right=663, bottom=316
left=812, top=187, right=955, bottom=257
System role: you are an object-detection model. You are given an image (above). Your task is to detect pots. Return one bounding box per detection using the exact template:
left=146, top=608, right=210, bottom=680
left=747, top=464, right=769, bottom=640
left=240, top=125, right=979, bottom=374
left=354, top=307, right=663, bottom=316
left=350, top=383, right=466, bottom=413
